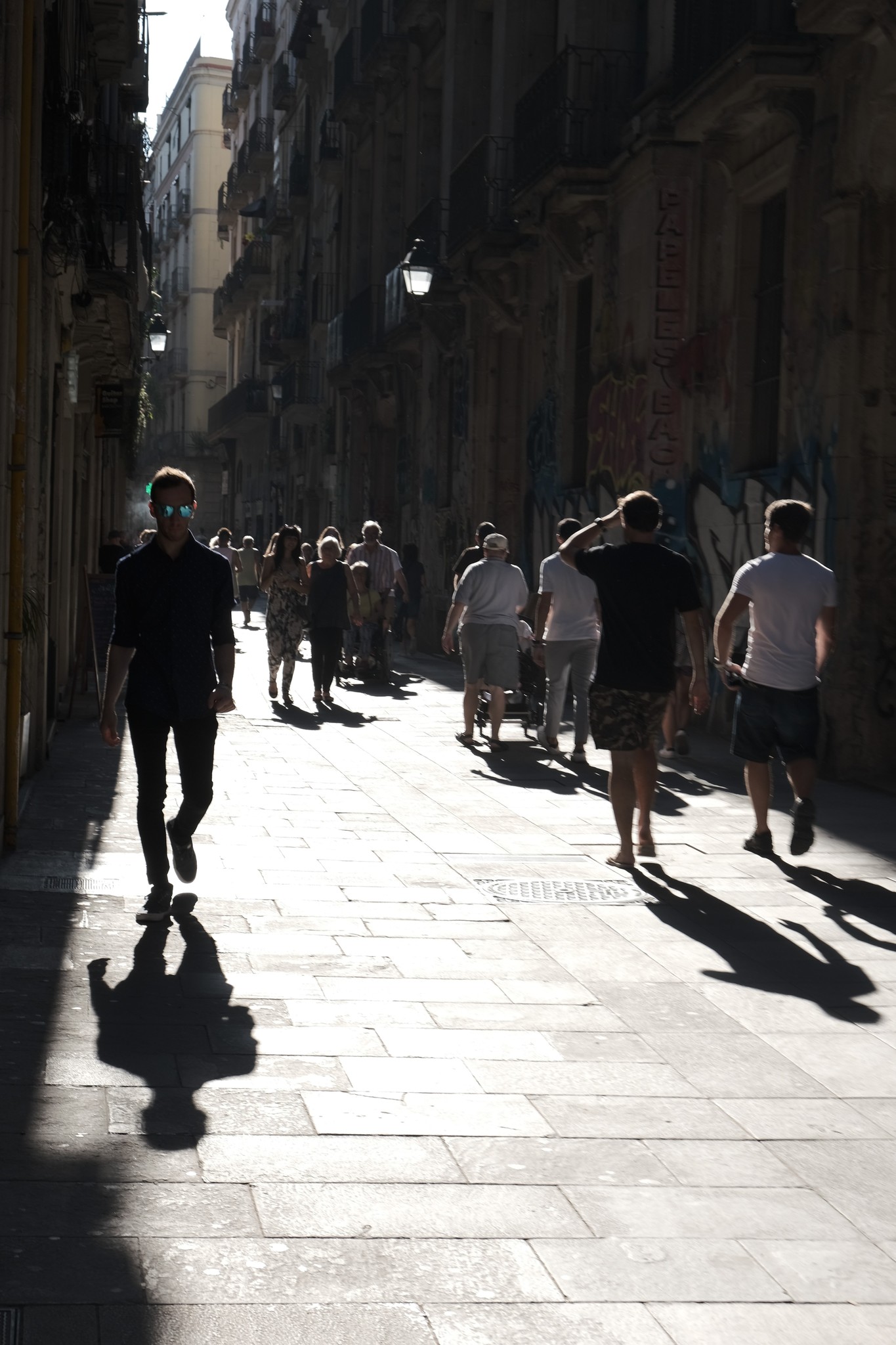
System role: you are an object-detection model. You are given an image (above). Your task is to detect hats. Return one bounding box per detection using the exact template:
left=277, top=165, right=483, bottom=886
left=482, top=533, right=510, bottom=553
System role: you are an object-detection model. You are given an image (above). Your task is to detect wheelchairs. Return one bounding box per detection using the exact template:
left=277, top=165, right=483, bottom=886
left=334, top=616, right=384, bottom=689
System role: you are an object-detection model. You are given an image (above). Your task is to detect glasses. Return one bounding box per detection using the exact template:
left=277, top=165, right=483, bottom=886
left=283, top=523, right=302, bottom=533
left=150, top=501, right=193, bottom=518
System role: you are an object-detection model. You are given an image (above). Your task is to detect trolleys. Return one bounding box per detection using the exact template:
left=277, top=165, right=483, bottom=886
left=474, top=615, right=539, bottom=736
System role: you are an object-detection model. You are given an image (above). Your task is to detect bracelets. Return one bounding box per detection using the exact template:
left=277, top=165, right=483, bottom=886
left=712, top=657, right=732, bottom=669
left=532, top=644, right=540, bottom=648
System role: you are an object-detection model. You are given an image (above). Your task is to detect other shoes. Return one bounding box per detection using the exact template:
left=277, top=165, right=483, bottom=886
left=243, top=608, right=250, bottom=626
left=282, top=693, right=293, bottom=704
left=268, top=680, right=278, bottom=698
left=312, top=691, right=334, bottom=703
left=744, top=829, right=773, bottom=855
left=166, top=818, right=197, bottom=884
left=658, top=747, right=675, bottom=758
left=537, top=725, right=560, bottom=755
left=136, top=882, right=173, bottom=922
left=674, top=728, right=689, bottom=755
left=790, top=797, right=815, bottom=855
left=571, top=750, right=587, bottom=763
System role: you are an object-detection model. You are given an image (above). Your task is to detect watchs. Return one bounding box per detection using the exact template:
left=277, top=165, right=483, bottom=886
left=531, top=638, right=540, bottom=644
left=218, top=683, right=232, bottom=689
left=593, top=516, right=607, bottom=532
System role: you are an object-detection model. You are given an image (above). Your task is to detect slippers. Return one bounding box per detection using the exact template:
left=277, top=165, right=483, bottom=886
left=604, top=855, right=634, bottom=869
left=637, top=844, right=656, bottom=857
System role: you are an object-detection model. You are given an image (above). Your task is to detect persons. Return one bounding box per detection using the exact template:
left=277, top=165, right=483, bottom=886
left=99, top=468, right=237, bottom=922
left=98, top=527, right=259, bottom=625
left=442, top=518, right=602, bottom=764
left=259, top=520, right=427, bottom=703
left=713, top=499, right=838, bottom=858
left=558, top=490, right=711, bottom=870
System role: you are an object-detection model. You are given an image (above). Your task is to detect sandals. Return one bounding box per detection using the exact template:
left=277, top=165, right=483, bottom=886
left=489, top=739, right=507, bottom=752
left=454, top=732, right=473, bottom=747
